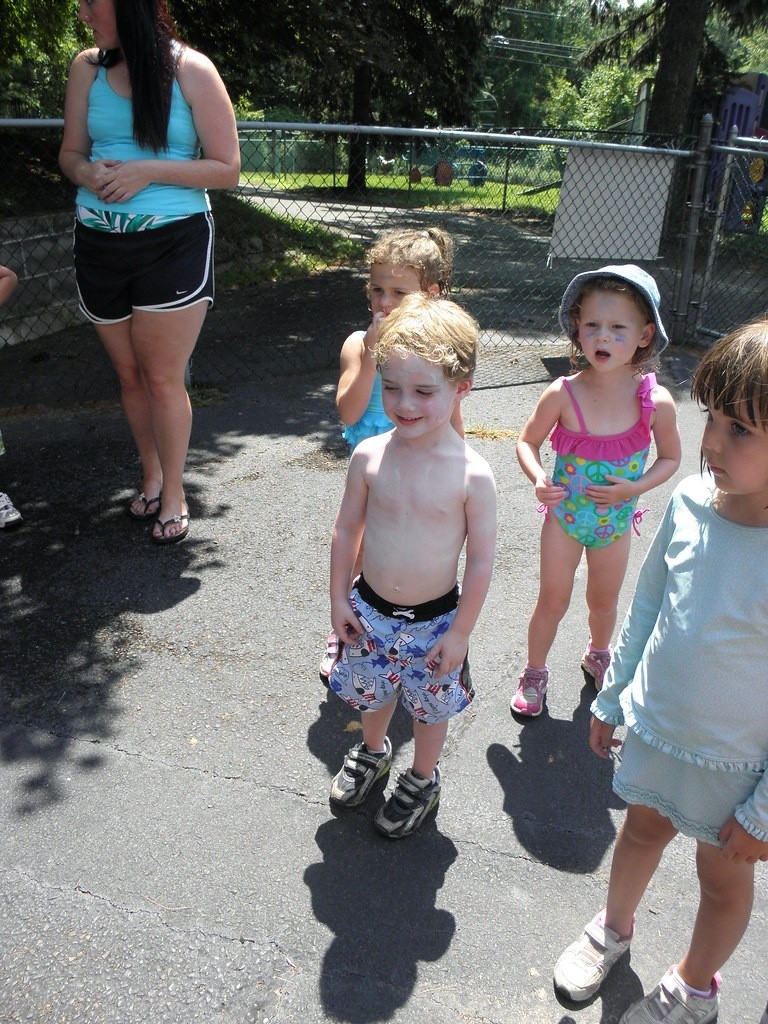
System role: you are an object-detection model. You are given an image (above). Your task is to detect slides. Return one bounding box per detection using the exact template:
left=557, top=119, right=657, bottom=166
left=517, top=179, right=563, bottom=197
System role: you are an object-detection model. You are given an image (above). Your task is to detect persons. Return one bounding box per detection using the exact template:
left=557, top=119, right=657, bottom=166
left=0, top=260, right=26, bottom=527
left=58, top=0, right=241, bottom=542
left=510, top=265, right=681, bottom=718
left=321, top=226, right=466, bottom=680
left=552, top=320, right=768, bottom=1024
left=329, top=291, right=498, bottom=839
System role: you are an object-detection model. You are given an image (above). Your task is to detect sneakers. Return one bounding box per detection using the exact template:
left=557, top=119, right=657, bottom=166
left=616, top=964, right=721, bottom=1024
left=579, top=634, right=615, bottom=693
left=328, top=734, right=392, bottom=810
left=372, top=760, right=443, bottom=838
left=0, top=492, right=23, bottom=527
left=553, top=908, right=638, bottom=1001
left=509, top=667, right=549, bottom=716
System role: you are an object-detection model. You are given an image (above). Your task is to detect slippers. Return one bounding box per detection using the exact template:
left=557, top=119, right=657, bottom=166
left=151, top=506, right=190, bottom=543
left=129, top=488, right=162, bottom=520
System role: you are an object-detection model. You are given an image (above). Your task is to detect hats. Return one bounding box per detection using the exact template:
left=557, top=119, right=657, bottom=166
left=557, top=263, right=669, bottom=366
